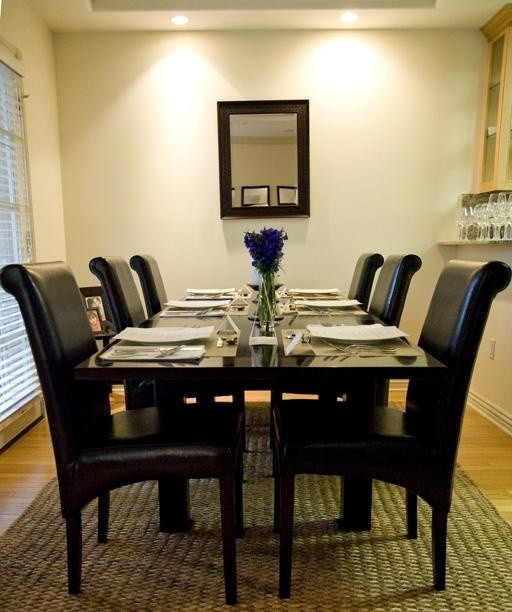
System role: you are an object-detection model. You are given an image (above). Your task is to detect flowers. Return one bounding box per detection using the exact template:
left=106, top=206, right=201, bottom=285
left=244, top=226, right=290, bottom=323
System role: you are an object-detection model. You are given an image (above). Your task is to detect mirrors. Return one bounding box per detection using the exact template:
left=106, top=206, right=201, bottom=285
left=216, top=99, right=311, bottom=219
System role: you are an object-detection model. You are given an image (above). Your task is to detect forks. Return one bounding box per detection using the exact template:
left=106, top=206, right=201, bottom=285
left=321, top=338, right=398, bottom=353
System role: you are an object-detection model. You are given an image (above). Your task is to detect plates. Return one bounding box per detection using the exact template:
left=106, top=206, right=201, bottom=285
left=306, top=323, right=409, bottom=344
left=186, top=288, right=236, bottom=297
left=111, top=325, right=215, bottom=346
left=99, top=345, right=208, bottom=361
left=289, top=288, right=340, bottom=295
left=294, top=298, right=362, bottom=309
left=163, top=300, right=230, bottom=310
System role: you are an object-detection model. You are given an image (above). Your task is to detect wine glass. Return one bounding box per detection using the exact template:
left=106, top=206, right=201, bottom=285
left=455, top=192, right=512, bottom=242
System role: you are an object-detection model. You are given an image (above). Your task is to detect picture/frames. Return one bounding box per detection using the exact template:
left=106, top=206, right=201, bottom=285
left=240, top=185, right=298, bottom=207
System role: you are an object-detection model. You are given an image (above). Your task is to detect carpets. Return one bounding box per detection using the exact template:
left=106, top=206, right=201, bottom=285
left=0, top=398, right=512, bottom=610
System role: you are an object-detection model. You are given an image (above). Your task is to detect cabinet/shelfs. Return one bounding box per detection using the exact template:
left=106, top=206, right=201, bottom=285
left=475, top=24, right=511, bottom=196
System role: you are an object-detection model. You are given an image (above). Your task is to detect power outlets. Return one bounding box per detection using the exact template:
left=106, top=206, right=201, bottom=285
left=488, top=338, right=495, bottom=360
